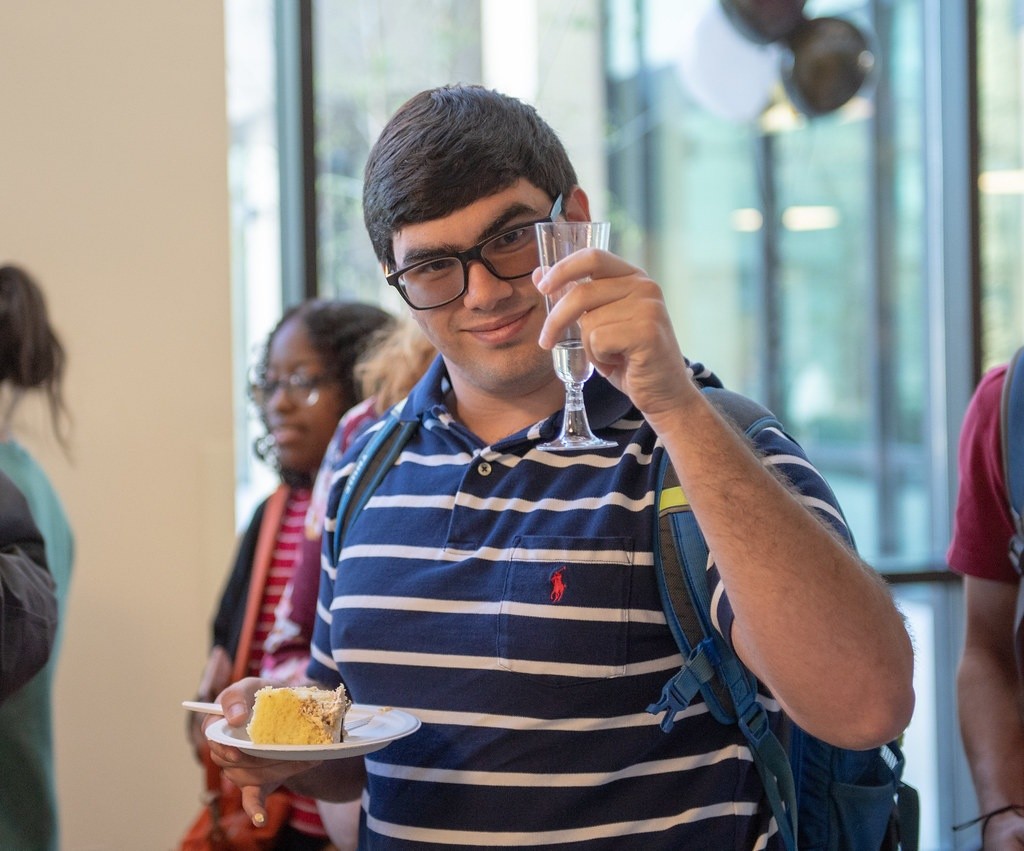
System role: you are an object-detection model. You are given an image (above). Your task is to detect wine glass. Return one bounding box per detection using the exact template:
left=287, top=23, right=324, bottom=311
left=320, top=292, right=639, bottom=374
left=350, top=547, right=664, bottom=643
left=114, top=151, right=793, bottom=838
left=535, top=222, right=619, bottom=452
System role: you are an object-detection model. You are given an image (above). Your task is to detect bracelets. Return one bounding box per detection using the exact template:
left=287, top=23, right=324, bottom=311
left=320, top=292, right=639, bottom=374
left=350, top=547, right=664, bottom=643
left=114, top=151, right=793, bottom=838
left=953, top=804, right=1024, bottom=846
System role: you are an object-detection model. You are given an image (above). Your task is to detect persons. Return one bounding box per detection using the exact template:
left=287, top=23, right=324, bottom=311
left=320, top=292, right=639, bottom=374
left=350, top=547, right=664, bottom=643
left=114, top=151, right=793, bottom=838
left=185, top=302, right=440, bottom=850
left=949, top=364, right=1024, bottom=851
left=0, top=262, right=74, bottom=851
left=200, top=83, right=914, bottom=851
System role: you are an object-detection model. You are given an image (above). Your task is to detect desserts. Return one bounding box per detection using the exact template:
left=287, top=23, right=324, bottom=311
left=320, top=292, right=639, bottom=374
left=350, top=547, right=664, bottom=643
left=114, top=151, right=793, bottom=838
left=247, top=681, right=351, bottom=746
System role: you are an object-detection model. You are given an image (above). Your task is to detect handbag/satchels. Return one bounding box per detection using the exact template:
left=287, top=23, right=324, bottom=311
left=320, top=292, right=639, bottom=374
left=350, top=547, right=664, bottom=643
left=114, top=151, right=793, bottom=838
left=182, top=788, right=293, bottom=851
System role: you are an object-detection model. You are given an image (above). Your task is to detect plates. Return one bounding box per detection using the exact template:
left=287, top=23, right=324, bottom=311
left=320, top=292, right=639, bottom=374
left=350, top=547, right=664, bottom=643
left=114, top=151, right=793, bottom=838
left=205, top=704, right=421, bottom=761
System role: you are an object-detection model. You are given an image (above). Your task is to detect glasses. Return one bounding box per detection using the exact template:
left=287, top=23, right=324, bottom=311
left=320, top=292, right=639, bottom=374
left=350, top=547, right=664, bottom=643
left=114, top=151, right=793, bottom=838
left=385, top=191, right=563, bottom=310
left=246, top=364, right=350, bottom=411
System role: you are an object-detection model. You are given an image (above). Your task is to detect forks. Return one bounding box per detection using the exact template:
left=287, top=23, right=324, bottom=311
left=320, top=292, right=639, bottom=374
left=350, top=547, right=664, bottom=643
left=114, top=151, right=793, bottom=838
left=182, top=701, right=375, bottom=730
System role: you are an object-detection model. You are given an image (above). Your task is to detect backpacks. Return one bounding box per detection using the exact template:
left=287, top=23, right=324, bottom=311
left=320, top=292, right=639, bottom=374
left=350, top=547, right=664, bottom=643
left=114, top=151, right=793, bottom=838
left=332, top=393, right=920, bottom=851
